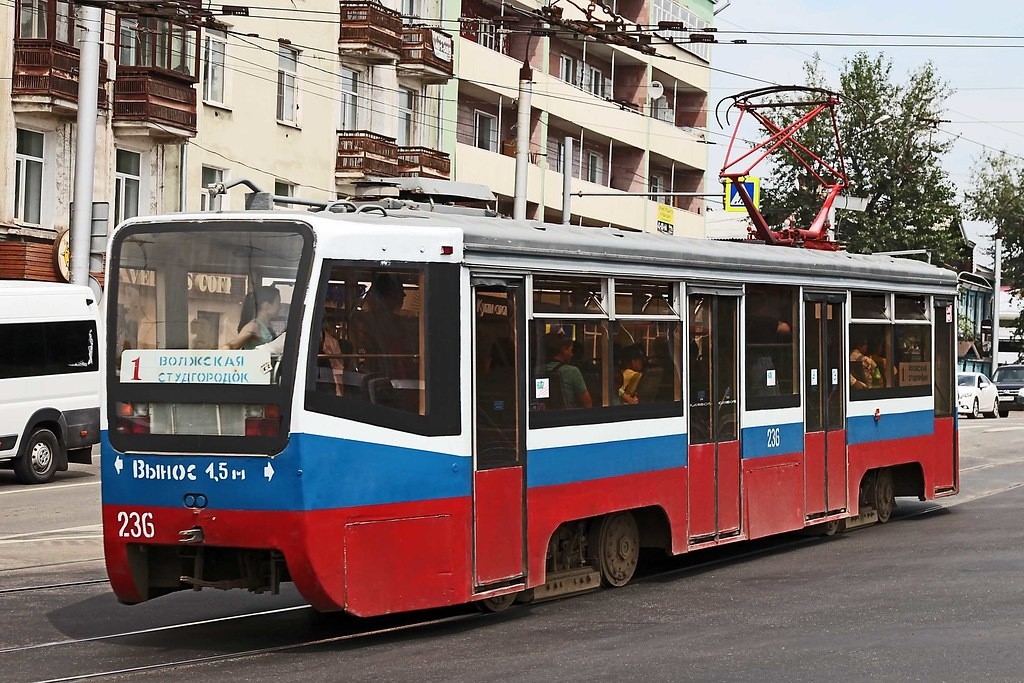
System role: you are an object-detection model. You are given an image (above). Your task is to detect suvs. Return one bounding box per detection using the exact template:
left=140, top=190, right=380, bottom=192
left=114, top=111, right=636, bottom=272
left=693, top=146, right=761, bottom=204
left=990, top=364, right=1024, bottom=418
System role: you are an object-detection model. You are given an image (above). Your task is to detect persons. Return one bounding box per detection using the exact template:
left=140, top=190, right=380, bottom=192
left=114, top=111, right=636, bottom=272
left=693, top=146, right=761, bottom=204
left=254, top=277, right=418, bottom=400
left=218, top=286, right=281, bottom=350
left=533, top=295, right=793, bottom=424
left=848, top=325, right=886, bottom=389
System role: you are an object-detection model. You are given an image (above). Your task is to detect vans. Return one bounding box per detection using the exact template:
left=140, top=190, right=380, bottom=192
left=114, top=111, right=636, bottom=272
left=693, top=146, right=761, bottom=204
left=0, top=278, right=103, bottom=485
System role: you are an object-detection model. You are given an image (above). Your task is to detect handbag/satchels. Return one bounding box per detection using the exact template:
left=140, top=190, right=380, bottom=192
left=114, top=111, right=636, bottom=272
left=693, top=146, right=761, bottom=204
left=314, top=331, right=336, bottom=408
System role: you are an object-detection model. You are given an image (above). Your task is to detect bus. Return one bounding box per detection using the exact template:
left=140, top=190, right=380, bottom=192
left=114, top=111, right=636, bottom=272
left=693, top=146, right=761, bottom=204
left=97, top=195, right=965, bottom=628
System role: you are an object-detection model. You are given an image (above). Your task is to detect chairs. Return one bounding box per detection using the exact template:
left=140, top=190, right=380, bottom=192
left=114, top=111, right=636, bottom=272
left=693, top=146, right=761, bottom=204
left=359, top=353, right=890, bottom=409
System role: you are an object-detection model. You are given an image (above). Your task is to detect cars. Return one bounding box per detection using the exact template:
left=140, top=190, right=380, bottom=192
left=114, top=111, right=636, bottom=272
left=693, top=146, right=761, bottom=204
left=955, top=371, right=1000, bottom=420
